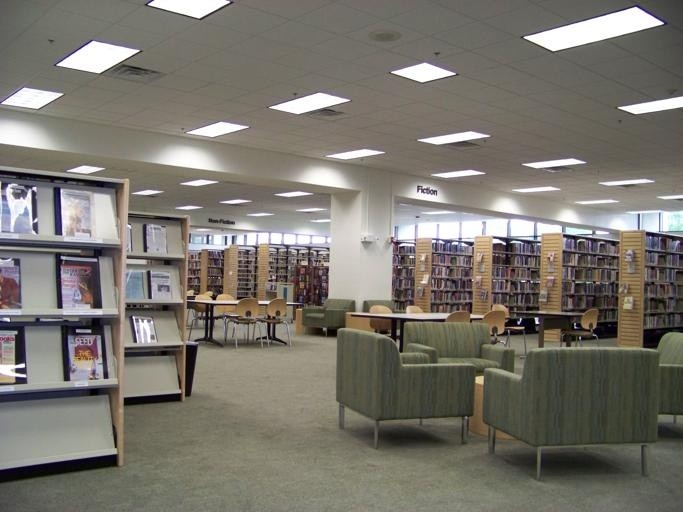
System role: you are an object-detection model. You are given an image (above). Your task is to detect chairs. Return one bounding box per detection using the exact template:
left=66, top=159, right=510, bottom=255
left=185, top=289, right=298, bottom=352
left=301, top=297, right=404, bottom=336
left=407, top=301, right=600, bottom=354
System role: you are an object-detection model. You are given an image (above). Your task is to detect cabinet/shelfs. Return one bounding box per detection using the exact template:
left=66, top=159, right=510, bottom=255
left=0, top=168, right=128, bottom=469
left=123, top=209, right=186, bottom=407
left=392, top=229, right=682, bottom=348
left=186, top=246, right=328, bottom=309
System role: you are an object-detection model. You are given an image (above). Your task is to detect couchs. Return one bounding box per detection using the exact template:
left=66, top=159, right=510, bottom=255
left=483, top=346, right=661, bottom=481
left=403, top=321, right=515, bottom=387
left=335, top=326, right=477, bottom=445
left=655, top=330, right=682, bottom=423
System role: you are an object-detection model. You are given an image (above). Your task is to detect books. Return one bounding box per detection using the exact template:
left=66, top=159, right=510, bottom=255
left=207, top=248, right=224, bottom=300
left=147, top=269, right=172, bottom=300
left=0, top=257, right=22, bottom=309
left=492, top=237, right=541, bottom=326
left=0, top=326, right=28, bottom=386
left=142, top=223, right=167, bottom=253
left=54, top=185, right=95, bottom=239
left=267, top=244, right=329, bottom=309
left=129, top=315, right=158, bottom=344
left=127, top=224, right=133, bottom=252
left=392, top=240, right=416, bottom=311
left=62, top=325, right=109, bottom=381
left=190, top=251, right=201, bottom=294
left=55, top=252, right=102, bottom=309
left=1, top=182, right=39, bottom=235
left=125, top=271, right=145, bottom=299
left=561, top=236, right=618, bottom=329
left=643, top=236, right=682, bottom=329
left=237, top=245, right=257, bottom=300
left=430, top=239, right=473, bottom=314
